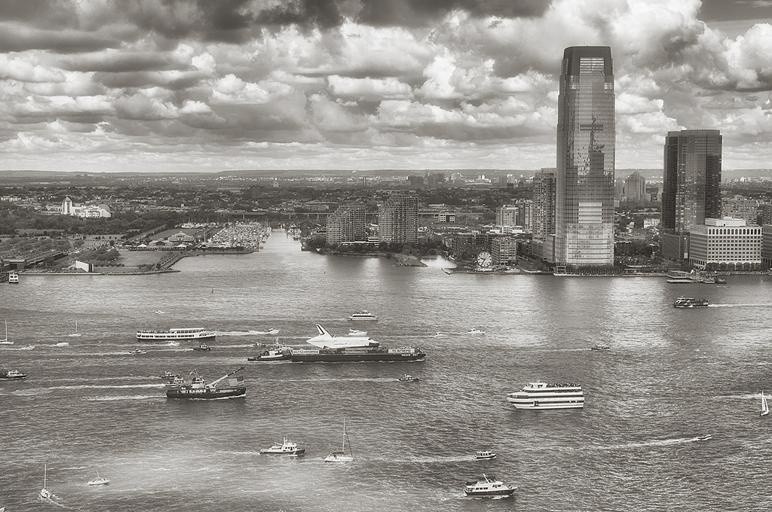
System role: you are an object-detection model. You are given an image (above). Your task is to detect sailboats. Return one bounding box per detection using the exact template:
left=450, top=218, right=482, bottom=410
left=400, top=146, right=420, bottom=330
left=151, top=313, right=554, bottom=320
left=760, top=390, right=770, bottom=418
left=323, top=417, right=356, bottom=463
left=1, top=319, right=15, bottom=346
left=38, top=463, right=52, bottom=502
left=69, top=320, right=81, bottom=337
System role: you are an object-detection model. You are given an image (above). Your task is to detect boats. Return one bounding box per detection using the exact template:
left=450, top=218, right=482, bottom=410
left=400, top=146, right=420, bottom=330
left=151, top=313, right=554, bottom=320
left=87, top=475, right=111, bottom=485
left=696, top=433, right=713, bottom=444
left=674, top=296, right=710, bottom=309
left=260, top=438, right=306, bottom=456
left=7, top=272, right=20, bottom=284
left=506, top=381, right=585, bottom=410
left=465, top=472, right=518, bottom=499
left=0, top=368, right=28, bottom=379
left=591, top=345, right=610, bottom=352
left=475, top=449, right=497, bottom=461
left=466, top=328, right=486, bottom=334
left=135, top=306, right=426, bottom=401
